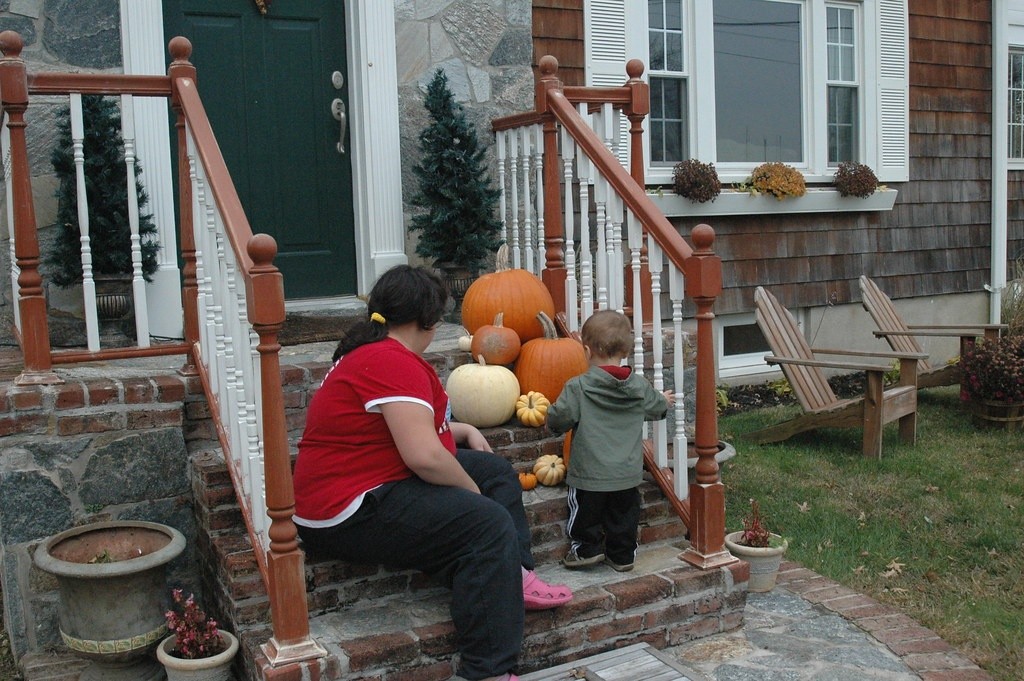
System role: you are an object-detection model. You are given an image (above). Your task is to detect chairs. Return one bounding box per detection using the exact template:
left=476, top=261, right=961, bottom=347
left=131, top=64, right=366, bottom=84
left=735, top=286, right=930, bottom=463
left=853, top=274, right=1009, bottom=399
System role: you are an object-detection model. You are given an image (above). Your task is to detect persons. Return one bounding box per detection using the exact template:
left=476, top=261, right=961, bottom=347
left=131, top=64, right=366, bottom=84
left=293, top=265, right=573, bottom=681
left=546, top=310, right=676, bottom=572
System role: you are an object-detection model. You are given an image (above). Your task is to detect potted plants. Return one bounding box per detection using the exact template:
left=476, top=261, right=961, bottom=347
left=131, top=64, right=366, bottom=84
left=45, top=94, right=164, bottom=348
left=405, top=67, right=505, bottom=318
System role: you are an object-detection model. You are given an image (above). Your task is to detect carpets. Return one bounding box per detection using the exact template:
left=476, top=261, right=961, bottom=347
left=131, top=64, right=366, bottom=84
left=278, top=314, right=369, bottom=345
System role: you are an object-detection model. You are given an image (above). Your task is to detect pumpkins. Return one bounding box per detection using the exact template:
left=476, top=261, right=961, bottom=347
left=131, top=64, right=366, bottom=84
left=514, top=312, right=588, bottom=404
left=515, top=391, right=551, bottom=427
left=457, top=328, right=474, bottom=351
left=446, top=354, right=521, bottom=428
left=533, top=454, right=566, bottom=486
left=471, top=313, right=521, bottom=365
left=562, top=429, right=572, bottom=468
left=519, top=471, right=537, bottom=490
left=462, top=243, right=555, bottom=345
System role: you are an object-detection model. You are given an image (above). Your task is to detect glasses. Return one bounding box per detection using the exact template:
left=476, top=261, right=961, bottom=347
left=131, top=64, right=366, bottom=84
left=436, top=318, right=445, bottom=328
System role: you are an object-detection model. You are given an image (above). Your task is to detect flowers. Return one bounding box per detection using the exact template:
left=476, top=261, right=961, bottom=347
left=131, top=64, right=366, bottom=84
left=831, top=162, right=879, bottom=200
left=159, top=587, right=225, bottom=658
left=672, top=158, right=722, bottom=204
left=946, top=335, right=1024, bottom=403
left=739, top=500, right=772, bottom=547
left=731, top=161, right=807, bottom=202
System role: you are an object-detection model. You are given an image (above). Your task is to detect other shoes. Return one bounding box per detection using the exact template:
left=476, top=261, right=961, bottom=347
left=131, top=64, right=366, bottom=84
left=603, top=551, right=634, bottom=572
left=563, top=544, right=606, bottom=567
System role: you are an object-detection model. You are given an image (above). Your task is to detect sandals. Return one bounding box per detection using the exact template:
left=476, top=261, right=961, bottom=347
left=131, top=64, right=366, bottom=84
left=508, top=673, right=522, bottom=681
left=520, top=569, right=572, bottom=612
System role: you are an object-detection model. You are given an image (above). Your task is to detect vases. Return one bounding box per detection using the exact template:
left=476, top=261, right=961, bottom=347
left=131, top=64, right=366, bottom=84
left=34, top=519, right=187, bottom=681
left=725, top=531, right=788, bottom=593
left=155, top=630, right=239, bottom=681
left=666, top=437, right=736, bottom=487
left=970, top=397, right=1024, bottom=432
